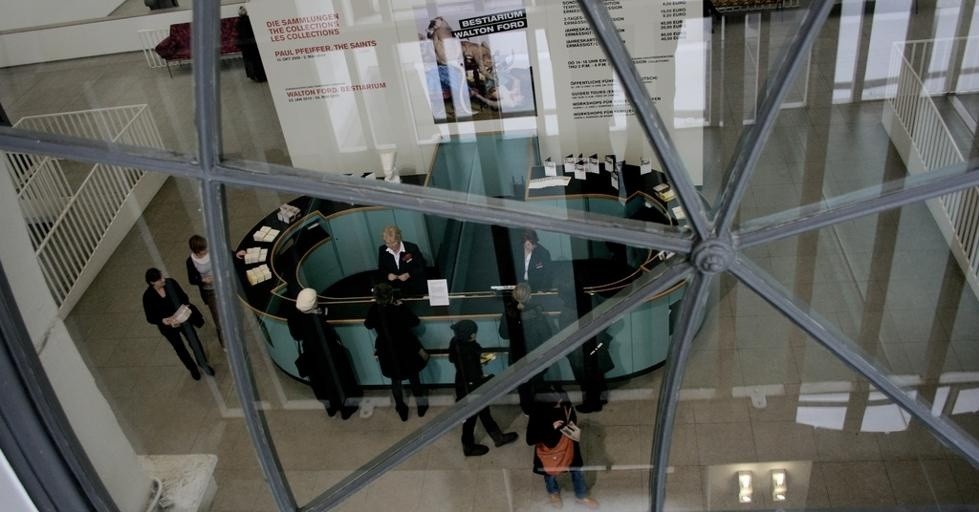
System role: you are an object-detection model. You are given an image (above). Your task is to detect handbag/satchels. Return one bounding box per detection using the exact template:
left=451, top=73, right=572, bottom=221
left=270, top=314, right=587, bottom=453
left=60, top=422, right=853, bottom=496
left=571, top=342, right=615, bottom=384
left=533, top=432, right=575, bottom=477
left=186, top=303, right=205, bottom=329
left=292, top=339, right=312, bottom=379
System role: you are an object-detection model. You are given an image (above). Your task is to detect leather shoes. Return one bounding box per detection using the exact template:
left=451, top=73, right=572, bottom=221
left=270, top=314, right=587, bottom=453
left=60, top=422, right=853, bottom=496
left=494, top=431, right=520, bottom=448
left=462, top=443, right=490, bottom=458
left=324, top=398, right=360, bottom=421
left=548, top=493, right=562, bottom=509
left=416, top=399, right=429, bottom=417
left=191, top=369, right=202, bottom=382
left=396, top=403, right=409, bottom=422
left=204, top=365, right=217, bottom=378
left=574, top=497, right=600, bottom=510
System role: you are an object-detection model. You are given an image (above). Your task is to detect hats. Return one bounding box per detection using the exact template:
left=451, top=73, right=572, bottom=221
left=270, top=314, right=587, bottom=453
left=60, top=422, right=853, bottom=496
left=296, top=287, right=318, bottom=312
left=449, top=319, right=479, bottom=341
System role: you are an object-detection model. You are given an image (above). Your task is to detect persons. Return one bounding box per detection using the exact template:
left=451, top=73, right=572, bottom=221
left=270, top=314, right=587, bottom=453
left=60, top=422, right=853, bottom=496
left=556, top=271, right=625, bottom=413
left=420, top=16, right=528, bottom=115
left=363, top=280, right=433, bottom=421
left=287, top=287, right=362, bottom=420
left=185, top=234, right=228, bottom=354
left=142, top=268, right=215, bottom=381
left=499, top=281, right=554, bottom=418
left=501, top=228, right=553, bottom=294
left=518, top=361, right=601, bottom=511
left=377, top=226, right=430, bottom=296
left=447, top=318, right=520, bottom=457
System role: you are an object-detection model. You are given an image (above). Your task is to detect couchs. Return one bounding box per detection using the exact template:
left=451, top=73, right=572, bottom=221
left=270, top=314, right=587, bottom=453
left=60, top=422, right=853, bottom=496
left=156, top=17, right=242, bottom=78
